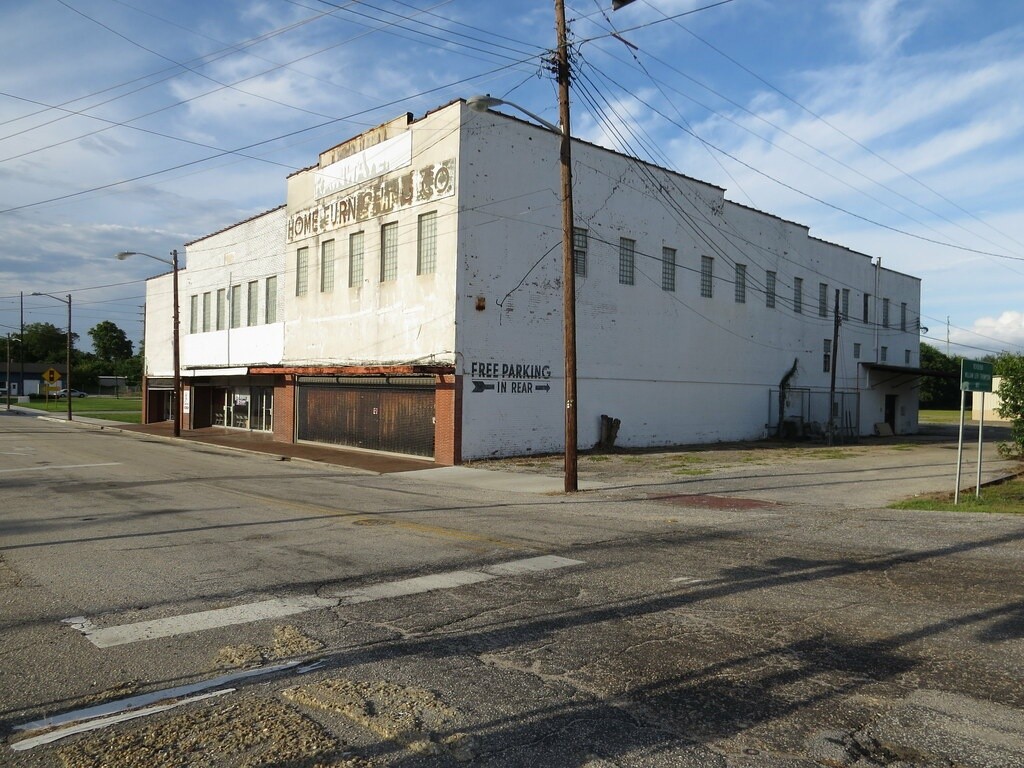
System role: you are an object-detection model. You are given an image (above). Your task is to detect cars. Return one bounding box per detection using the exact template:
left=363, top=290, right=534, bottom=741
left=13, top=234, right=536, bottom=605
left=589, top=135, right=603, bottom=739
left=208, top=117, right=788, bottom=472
left=59, top=388, right=89, bottom=399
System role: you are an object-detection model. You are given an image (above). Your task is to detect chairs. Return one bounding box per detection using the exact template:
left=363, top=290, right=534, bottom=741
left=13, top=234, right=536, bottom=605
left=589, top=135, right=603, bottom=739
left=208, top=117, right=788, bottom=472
left=215, top=414, right=248, bottom=428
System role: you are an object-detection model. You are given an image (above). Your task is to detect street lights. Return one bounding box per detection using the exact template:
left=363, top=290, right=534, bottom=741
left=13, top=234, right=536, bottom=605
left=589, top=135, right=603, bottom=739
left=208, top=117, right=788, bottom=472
left=464, top=92, right=579, bottom=494
left=114, top=247, right=182, bottom=438
left=12, top=337, right=25, bottom=397
left=30, top=292, right=73, bottom=422
